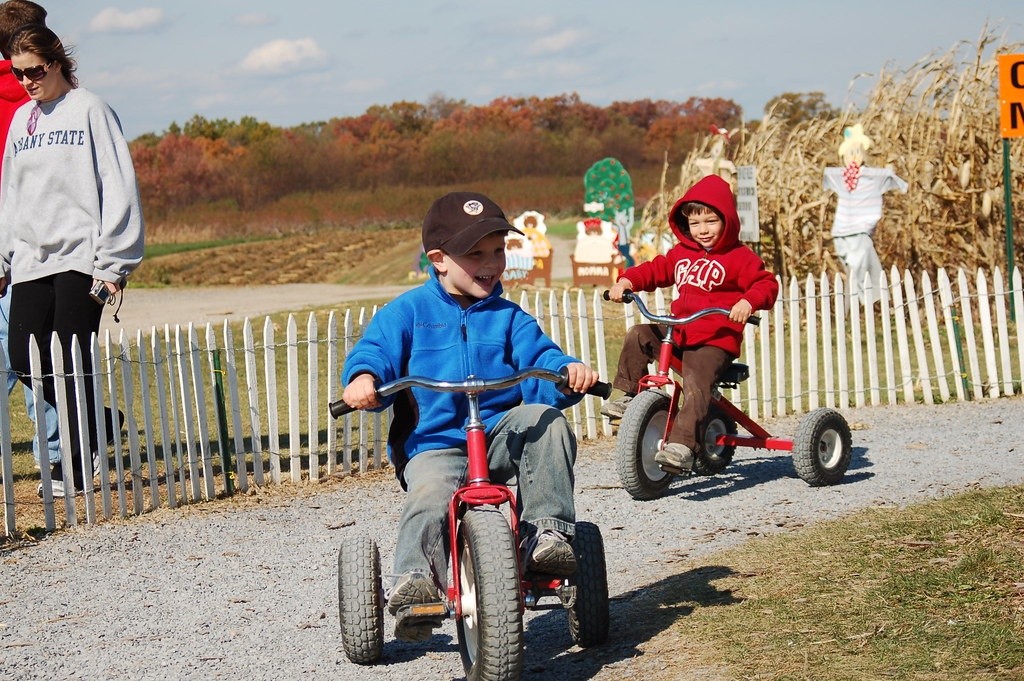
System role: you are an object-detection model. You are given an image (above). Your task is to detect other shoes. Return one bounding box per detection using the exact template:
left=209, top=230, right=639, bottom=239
left=37, top=457, right=94, bottom=497
left=91, top=449, right=101, bottom=475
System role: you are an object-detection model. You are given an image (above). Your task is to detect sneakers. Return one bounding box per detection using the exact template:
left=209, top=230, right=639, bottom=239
left=654, top=442, right=694, bottom=471
left=384, top=571, right=439, bottom=643
left=518, top=528, right=578, bottom=581
left=600, top=393, right=636, bottom=417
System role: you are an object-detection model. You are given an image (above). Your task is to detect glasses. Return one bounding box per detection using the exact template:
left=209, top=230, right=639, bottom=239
left=10, top=62, right=52, bottom=82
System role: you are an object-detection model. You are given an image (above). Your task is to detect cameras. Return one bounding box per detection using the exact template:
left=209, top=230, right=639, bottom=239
left=89, top=280, right=111, bottom=305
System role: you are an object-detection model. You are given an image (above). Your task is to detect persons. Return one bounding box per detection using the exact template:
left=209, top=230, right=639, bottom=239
left=602, top=174, right=778, bottom=469
left=1, top=25, right=146, bottom=499
left=1, top=0, right=101, bottom=478
left=343, top=193, right=599, bottom=646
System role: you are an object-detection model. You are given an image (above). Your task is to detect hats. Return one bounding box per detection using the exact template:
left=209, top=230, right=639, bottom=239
left=421, top=191, right=525, bottom=256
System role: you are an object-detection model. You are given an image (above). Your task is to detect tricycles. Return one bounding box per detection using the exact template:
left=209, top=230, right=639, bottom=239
left=327, top=366, right=611, bottom=680
left=601, top=290, right=852, bottom=502
